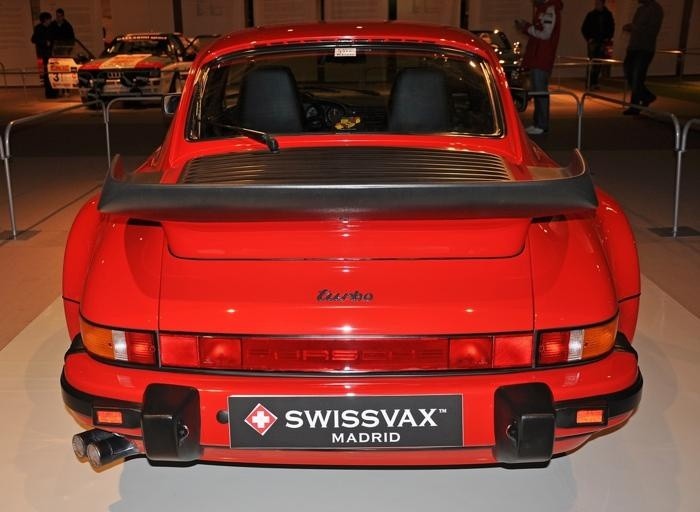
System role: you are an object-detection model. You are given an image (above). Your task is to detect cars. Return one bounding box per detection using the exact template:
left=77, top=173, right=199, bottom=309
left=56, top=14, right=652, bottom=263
left=470, top=30, right=528, bottom=114
left=46, top=29, right=224, bottom=110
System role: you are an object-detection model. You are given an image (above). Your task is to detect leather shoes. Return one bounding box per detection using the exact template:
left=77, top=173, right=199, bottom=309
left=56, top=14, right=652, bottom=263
left=622, top=106, right=641, bottom=115
left=641, top=94, right=657, bottom=108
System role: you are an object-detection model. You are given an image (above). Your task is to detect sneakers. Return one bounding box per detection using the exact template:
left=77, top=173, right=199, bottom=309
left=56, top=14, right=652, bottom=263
left=525, top=124, right=549, bottom=137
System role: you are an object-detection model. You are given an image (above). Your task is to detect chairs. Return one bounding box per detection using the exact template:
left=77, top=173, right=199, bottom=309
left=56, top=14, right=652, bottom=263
left=385, top=66, right=457, bottom=134
left=234, top=62, right=308, bottom=131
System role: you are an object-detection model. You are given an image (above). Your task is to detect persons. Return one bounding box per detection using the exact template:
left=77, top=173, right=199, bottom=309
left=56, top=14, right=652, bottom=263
left=621, top=0, right=664, bottom=116
left=30, top=12, right=57, bottom=98
left=581, top=1, right=617, bottom=92
left=513, top=0, right=564, bottom=136
left=53, top=8, right=75, bottom=95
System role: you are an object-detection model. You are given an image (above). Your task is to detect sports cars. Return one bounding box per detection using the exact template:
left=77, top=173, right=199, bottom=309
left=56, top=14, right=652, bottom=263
left=57, top=22, right=645, bottom=473
